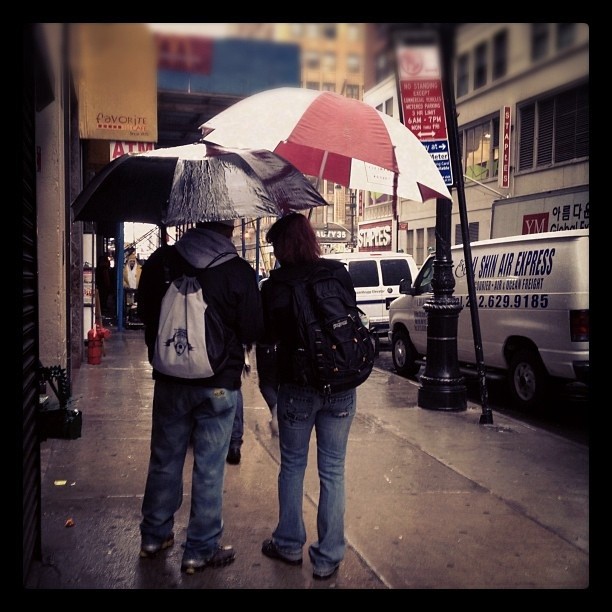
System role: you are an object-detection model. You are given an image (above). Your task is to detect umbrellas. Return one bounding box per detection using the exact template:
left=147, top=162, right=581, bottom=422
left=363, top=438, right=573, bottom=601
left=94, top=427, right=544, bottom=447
left=68, top=142, right=330, bottom=229
left=196, top=85, right=455, bottom=222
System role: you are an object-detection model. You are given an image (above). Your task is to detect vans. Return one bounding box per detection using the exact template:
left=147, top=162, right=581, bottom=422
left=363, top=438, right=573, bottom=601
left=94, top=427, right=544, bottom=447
left=386, top=229, right=589, bottom=402
left=312, top=251, right=418, bottom=351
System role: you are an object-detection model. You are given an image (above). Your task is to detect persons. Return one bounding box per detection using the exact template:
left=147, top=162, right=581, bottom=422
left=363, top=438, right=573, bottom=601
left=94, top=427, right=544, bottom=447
left=254, top=211, right=375, bottom=580
left=224, top=388, right=244, bottom=466
left=134, top=218, right=261, bottom=575
left=256, top=343, right=279, bottom=436
left=123, top=256, right=141, bottom=289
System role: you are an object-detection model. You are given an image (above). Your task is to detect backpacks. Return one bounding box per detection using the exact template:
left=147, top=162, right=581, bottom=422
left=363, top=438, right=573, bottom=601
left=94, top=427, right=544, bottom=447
left=153, top=251, right=239, bottom=379
left=279, top=264, right=374, bottom=393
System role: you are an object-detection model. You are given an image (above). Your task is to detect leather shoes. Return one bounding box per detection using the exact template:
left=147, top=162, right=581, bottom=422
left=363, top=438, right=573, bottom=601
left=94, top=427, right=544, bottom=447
left=227, top=447, right=240, bottom=464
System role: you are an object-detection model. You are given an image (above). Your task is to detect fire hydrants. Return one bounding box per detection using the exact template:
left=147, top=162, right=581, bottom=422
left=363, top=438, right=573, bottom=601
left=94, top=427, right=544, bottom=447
left=87, top=323, right=112, bottom=365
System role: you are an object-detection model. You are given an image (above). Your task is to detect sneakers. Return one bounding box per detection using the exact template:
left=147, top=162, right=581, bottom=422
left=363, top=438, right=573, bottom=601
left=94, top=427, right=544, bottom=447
left=262, top=538, right=303, bottom=565
left=182, top=543, right=235, bottom=574
left=140, top=531, right=174, bottom=557
left=313, top=562, right=340, bottom=579
